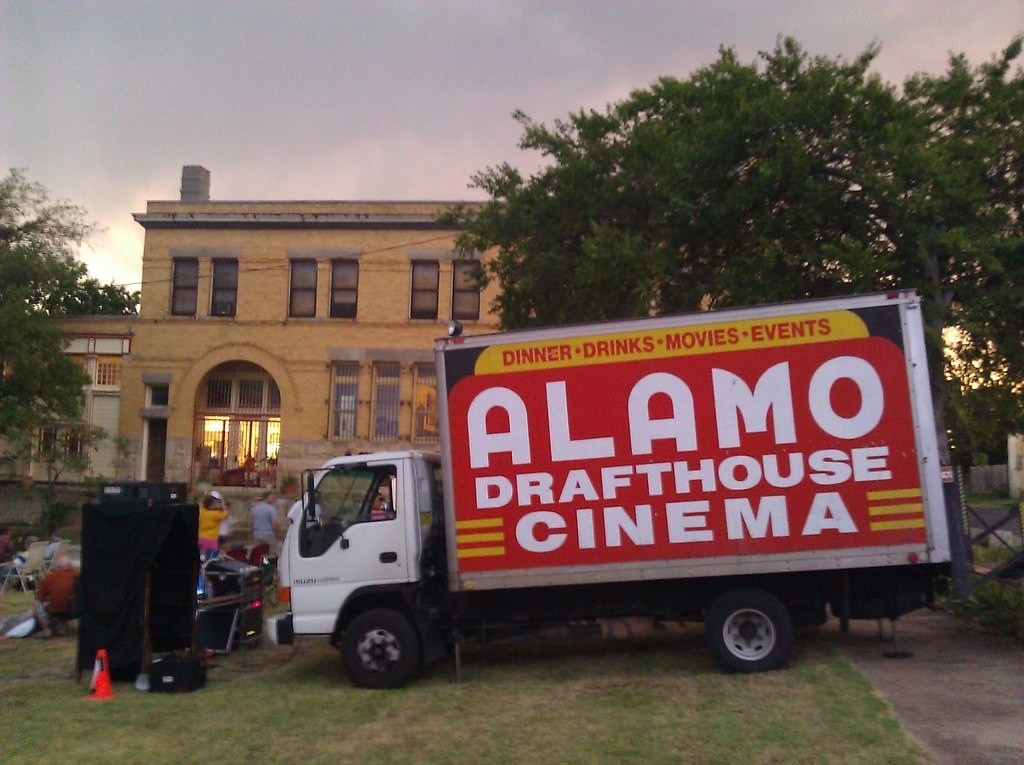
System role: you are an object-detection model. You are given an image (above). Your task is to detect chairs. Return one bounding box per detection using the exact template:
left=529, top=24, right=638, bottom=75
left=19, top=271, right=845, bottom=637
left=46, top=575, right=80, bottom=641
left=1, top=541, right=49, bottom=599
left=259, top=555, right=279, bottom=606
left=37, top=540, right=70, bottom=578
left=226, top=544, right=270, bottom=567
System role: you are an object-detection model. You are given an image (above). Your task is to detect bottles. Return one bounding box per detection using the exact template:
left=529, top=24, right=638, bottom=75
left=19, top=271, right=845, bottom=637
left=205, top=579, right=213, bottom=600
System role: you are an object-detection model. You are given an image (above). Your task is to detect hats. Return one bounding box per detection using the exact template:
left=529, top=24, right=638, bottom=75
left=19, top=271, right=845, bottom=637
left=52, top=529, right=66, bottom=538
left=263, top=488, right=276, bottom=497
left=210, top=490, right=225, bottom=500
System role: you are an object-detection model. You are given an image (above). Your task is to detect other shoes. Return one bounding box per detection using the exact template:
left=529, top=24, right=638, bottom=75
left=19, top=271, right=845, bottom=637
left=32, top=629, right=52, bottom=640
left=55, top=628, right=66, bottom=637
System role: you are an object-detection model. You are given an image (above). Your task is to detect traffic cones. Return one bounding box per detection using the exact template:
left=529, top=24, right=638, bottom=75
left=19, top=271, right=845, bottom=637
left=82, top=648, right=121, bottom=701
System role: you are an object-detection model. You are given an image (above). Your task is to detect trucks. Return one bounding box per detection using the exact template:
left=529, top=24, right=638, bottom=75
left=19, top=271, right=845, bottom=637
left=264, top=287, right=955, bottom=694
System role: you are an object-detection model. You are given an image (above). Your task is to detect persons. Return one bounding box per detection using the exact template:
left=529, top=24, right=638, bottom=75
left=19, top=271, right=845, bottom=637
left=288, top=490, right=323, bottom=529
left=199, top=492, right=228, bottom=599
left=251, top=491, right=289, bottom=563
left=0, top=528, right=85, bottom=638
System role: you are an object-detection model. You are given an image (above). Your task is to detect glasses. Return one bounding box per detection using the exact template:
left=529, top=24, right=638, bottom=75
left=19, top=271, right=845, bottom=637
left=378, top=496, right=385, bottom=502
